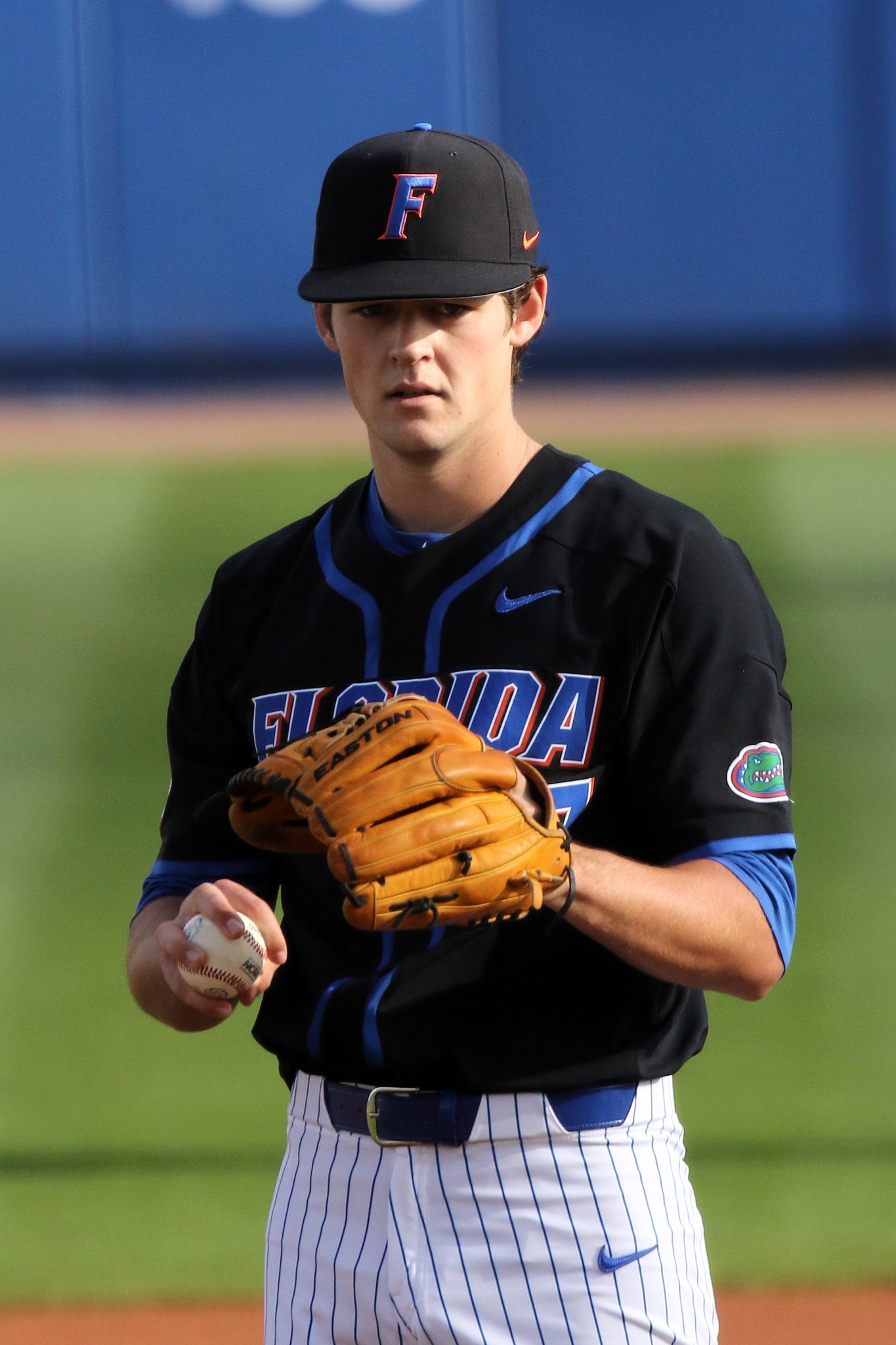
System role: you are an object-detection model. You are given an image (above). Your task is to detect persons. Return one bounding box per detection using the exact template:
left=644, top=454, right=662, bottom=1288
left=122, top=121, right=798, bottom=1345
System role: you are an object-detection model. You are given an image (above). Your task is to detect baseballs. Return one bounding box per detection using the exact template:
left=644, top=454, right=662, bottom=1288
left=176, top=912, right=267, bottom=1000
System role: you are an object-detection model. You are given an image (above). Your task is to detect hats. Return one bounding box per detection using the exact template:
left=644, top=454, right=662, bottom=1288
left=297, top=123, right=537, bottom=301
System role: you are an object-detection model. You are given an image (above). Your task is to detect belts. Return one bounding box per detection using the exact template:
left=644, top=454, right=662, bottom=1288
left=322, top=1081, right=635, bottom=1144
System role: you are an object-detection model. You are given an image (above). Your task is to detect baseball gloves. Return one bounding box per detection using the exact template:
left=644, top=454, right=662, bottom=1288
left=226, top=692, right=577, bottom=941
